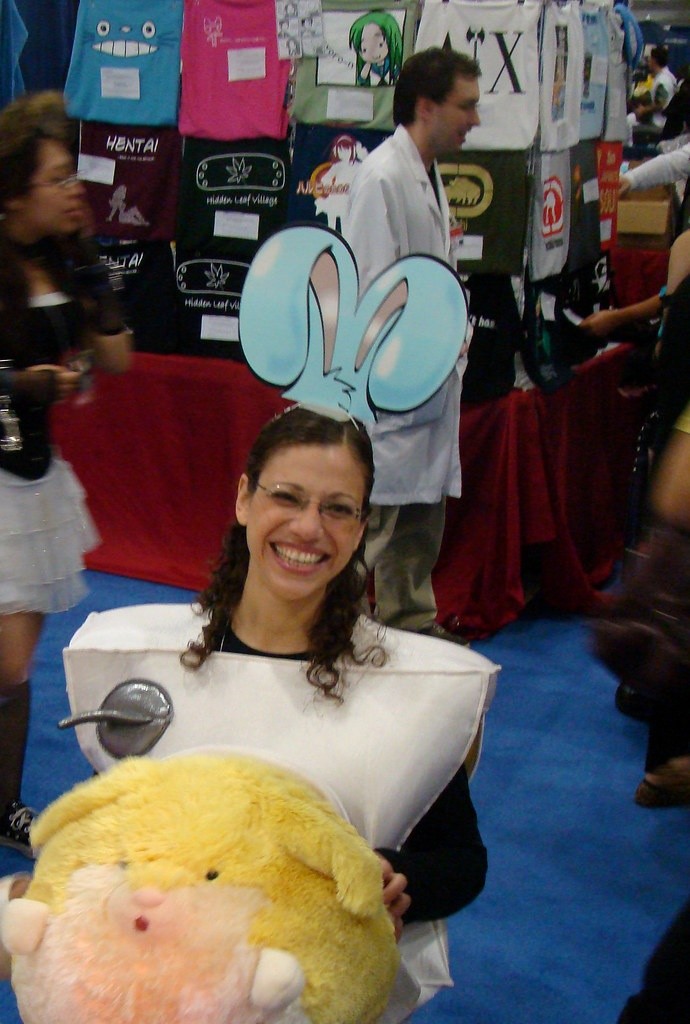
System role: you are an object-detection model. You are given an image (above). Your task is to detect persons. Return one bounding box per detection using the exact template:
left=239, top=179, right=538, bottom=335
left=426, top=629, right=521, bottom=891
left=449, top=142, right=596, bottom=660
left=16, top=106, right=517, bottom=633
left=58, top=407, right=503, bottom=1024
left=0, top=114, right=131, bottom=861
left=612, top=405, right=690, bottom=1024
left=342, top=50, right=481, bottom=649
left=576, top=45, right=690, bottom=809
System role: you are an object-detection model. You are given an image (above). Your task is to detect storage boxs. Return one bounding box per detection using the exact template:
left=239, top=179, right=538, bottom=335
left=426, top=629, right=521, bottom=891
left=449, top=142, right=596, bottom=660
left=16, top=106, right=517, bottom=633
left=616, top=161, right=679, bottom=249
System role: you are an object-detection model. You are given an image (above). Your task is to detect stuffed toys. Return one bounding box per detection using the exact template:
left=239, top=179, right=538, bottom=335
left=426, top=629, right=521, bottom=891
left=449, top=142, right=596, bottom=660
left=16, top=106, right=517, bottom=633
left=0, top=744, right=398, bottom=1024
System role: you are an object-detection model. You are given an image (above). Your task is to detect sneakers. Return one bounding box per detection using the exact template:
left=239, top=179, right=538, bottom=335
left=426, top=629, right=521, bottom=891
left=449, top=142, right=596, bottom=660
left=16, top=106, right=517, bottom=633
left=0, top=799, right=40, bottom=860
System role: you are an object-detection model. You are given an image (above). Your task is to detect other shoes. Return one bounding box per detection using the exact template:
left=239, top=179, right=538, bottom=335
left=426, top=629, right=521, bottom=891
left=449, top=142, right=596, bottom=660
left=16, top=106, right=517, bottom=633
left=423, top=623, right=470, bottom=648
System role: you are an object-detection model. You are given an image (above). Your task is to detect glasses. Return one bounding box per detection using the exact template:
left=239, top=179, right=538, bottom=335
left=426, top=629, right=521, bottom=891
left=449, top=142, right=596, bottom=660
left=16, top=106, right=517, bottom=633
left=251, top=478, right=361, bottom=528
left=28, top=171, right=81, bottom=191
